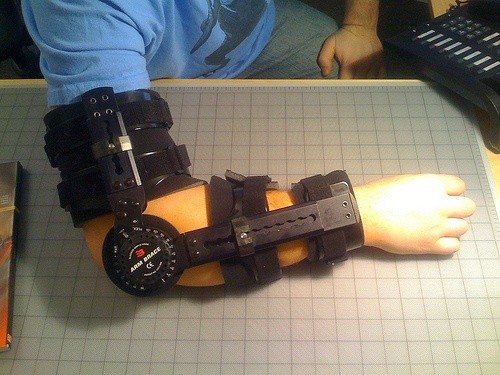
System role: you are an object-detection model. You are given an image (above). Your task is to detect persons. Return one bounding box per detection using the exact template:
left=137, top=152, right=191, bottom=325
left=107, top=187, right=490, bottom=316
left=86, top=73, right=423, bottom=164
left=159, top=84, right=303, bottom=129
left=20, top=1, right=474, bottom=298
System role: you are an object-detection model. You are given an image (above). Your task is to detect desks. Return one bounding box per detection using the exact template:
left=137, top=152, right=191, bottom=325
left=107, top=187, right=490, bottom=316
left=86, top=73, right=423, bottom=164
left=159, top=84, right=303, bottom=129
left=1, top=78, right=500, bottom=375
